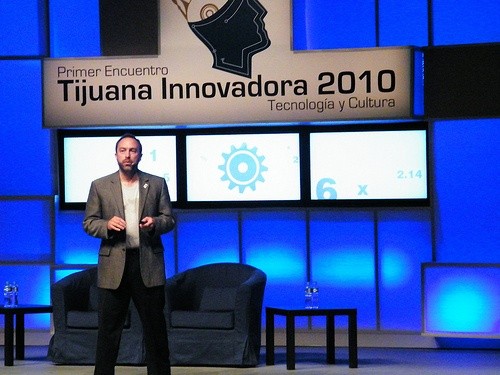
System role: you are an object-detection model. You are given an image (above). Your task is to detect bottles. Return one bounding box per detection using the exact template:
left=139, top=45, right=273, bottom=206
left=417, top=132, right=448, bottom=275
left=304, top=281, right=312, bottom=309
left=310, top=281, right=319, bottom=309
left=3, top=281, right=10, bottom=308
left=10, top=281, right=18, bottom=307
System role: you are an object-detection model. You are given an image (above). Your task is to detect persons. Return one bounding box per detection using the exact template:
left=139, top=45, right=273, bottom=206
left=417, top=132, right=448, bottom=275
left=82, top=133, right=176, bottom=375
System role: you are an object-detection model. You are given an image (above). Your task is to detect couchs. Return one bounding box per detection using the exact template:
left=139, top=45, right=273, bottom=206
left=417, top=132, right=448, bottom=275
left=46, top=266, right=146, bottom=365
left=164, top=262, right=267, bottom=367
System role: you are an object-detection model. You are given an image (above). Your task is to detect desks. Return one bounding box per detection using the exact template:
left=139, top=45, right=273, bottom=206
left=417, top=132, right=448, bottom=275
left=265, top=305, right=359, bottom=370
left=0, top=303, right=53, bottom=366
